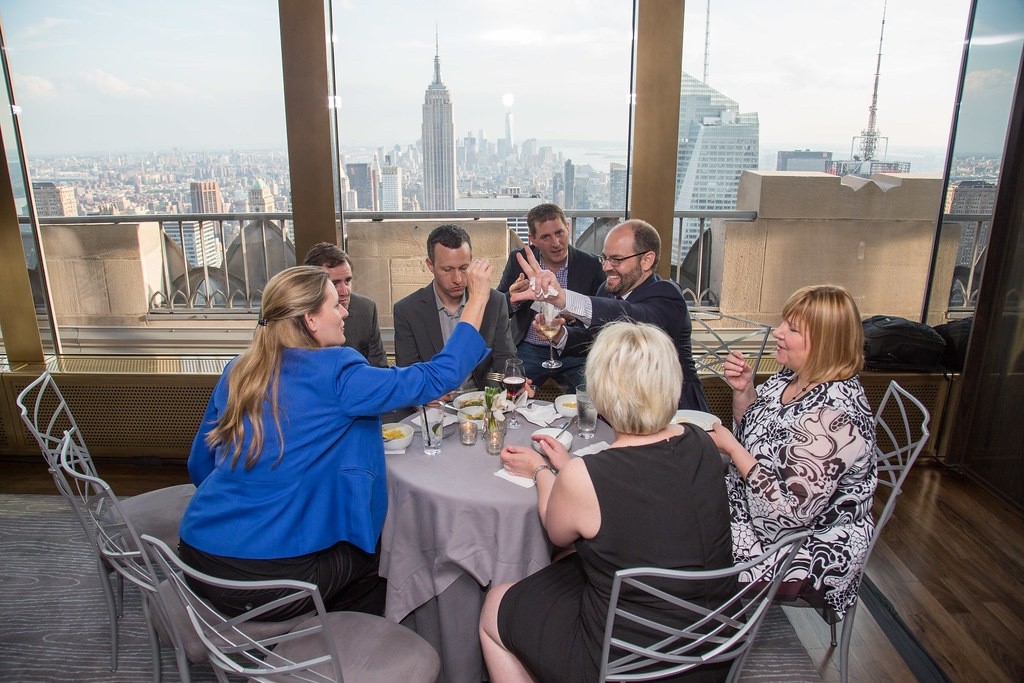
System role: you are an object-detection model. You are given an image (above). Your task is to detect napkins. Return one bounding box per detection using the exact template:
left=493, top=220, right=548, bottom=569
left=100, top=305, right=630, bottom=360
left=516, top=404, right=563, bottom=427
left=412, top=407, right=460, bottom=430
left=530, top=301, right=560, bottom=318
left=530, top=276, right=559, bottom=299
left=572, top=442, right=610, bottom=456
left=494, top=468, right=556, bottom=488
left=383, top=448, right=408, bottom=454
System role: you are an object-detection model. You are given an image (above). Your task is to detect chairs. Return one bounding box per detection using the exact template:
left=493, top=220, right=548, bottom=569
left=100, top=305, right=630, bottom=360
left=60, top=431, right=318, bottom=682
left=729, top=380, right=930, bottom=683
left=141, top=533, right=443, bottom=683
left=599, top=532, right=808, bottom=683
left=688, top=309, right=772, bottom=421
left=16, top=371, right=196, bottom=674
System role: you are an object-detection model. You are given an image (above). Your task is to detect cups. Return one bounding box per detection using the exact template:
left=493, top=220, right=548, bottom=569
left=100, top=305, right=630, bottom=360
left=575, top=384, right=597, bottom=439
left=460, top=422, right=477, bottom=445
left=485, top=427, right=505, bottom=454
left=496, top=416, right=507, bottom=437
left=419, top=398, right=444, bottom=454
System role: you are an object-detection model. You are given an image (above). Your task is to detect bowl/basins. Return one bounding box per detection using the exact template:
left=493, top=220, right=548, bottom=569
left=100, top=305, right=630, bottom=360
left=531, top=428, right=573, bottom=458
left=555, top=394, right=579, bottom=418
left=380, top=423, right=414, bottom=451
left=457, top=406, right=485, bottom=432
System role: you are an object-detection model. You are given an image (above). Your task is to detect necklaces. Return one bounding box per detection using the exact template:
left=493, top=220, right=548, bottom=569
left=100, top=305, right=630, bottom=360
left=617, top=428, right=665, bottom=436
left=738, top=375, right=812, bottom=446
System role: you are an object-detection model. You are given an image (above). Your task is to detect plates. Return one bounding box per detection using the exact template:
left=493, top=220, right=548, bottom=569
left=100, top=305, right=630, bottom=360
left=669, top=409, right=722, bottom=432
left=453, top=391, right=486, bottom=410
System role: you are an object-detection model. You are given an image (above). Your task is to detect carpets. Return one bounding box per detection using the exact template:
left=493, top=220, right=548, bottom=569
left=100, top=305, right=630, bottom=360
left=733, top=593, right=822, bottom=683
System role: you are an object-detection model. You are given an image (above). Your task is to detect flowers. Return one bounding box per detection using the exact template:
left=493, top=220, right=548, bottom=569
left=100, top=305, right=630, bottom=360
left=481, top=386, right=508, bottom=434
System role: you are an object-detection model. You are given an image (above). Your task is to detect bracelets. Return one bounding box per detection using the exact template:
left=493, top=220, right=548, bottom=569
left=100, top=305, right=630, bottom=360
left=532, top=465, right=554, bottom=480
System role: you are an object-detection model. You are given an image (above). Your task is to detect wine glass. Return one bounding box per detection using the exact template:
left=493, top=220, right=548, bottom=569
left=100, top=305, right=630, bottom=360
left=539, top=301, right=562, bottom=369
left=501, top=359, right=526, bottom=430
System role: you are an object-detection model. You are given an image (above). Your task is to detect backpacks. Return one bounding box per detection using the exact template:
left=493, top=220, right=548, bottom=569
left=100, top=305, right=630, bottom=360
left=862, top=315, right=948, bottom=373
left=935, top=316, right=972, bottom=372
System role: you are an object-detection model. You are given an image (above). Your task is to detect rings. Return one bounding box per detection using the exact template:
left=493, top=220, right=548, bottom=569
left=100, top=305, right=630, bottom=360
left=475, top=262, right=479, bottom=263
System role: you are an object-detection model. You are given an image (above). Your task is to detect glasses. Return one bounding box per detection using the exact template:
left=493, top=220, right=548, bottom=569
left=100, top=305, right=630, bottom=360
left=597, top=250, right=650, bottom=266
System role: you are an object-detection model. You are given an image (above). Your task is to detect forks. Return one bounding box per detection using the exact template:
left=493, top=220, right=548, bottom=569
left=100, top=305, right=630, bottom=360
left=414, top=430, right=455, bottom=438
left=486, top=372, right=539, bottom=392
left=545, top=422, right=567, bottom=429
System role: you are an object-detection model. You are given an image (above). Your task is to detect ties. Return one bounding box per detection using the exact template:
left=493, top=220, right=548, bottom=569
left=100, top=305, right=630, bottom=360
left=615, top=296, right=624, bottom=300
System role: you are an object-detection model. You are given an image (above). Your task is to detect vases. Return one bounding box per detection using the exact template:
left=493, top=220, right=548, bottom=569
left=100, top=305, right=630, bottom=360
left=481, top=413, right=506, bottom=440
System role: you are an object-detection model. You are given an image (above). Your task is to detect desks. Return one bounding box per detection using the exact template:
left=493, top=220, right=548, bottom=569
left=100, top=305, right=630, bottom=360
left=374, top=399, right=618, bottom=683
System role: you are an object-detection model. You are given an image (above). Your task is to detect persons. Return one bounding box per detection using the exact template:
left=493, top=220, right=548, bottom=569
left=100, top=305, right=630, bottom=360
left=706, top=284, right=879, bottom=624
left=510, top=219, right=710, bottom=412
left=496, top=203, right=607, bottom=388
left=177, top=259, right=494, bottom=622
left=303, top=242, right=390, bottom=368
left=478, top=320, right=747, bottom=683
left=393, top=224, right=535, bottom=407
